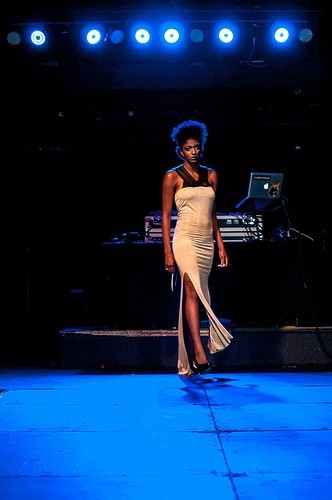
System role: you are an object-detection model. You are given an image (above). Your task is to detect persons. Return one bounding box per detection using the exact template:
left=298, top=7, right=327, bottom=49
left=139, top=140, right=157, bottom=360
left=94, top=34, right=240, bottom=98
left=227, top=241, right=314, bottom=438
left=162, top=121, right=233, bottom=375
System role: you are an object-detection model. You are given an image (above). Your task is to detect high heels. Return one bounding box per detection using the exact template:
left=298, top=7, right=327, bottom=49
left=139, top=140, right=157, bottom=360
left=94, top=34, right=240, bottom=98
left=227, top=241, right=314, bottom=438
left=190, top=352, right=211, bottom=376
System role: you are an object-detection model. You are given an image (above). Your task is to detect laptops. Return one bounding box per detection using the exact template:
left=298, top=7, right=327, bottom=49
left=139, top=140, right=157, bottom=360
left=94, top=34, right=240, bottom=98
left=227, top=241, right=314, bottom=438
left=235, top=172, right=283, bottom=212
